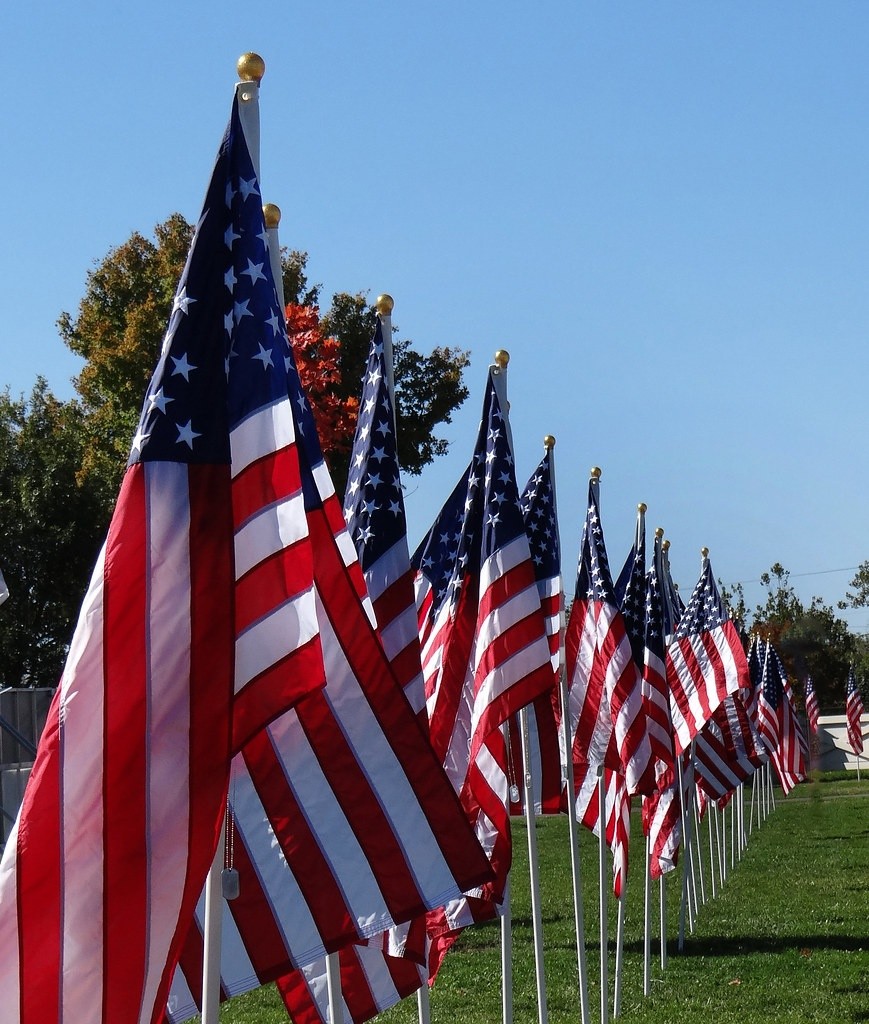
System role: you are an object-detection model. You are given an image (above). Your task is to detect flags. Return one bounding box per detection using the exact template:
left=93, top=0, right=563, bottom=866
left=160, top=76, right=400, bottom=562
left=1, top=56, right=863, bottom=1024
